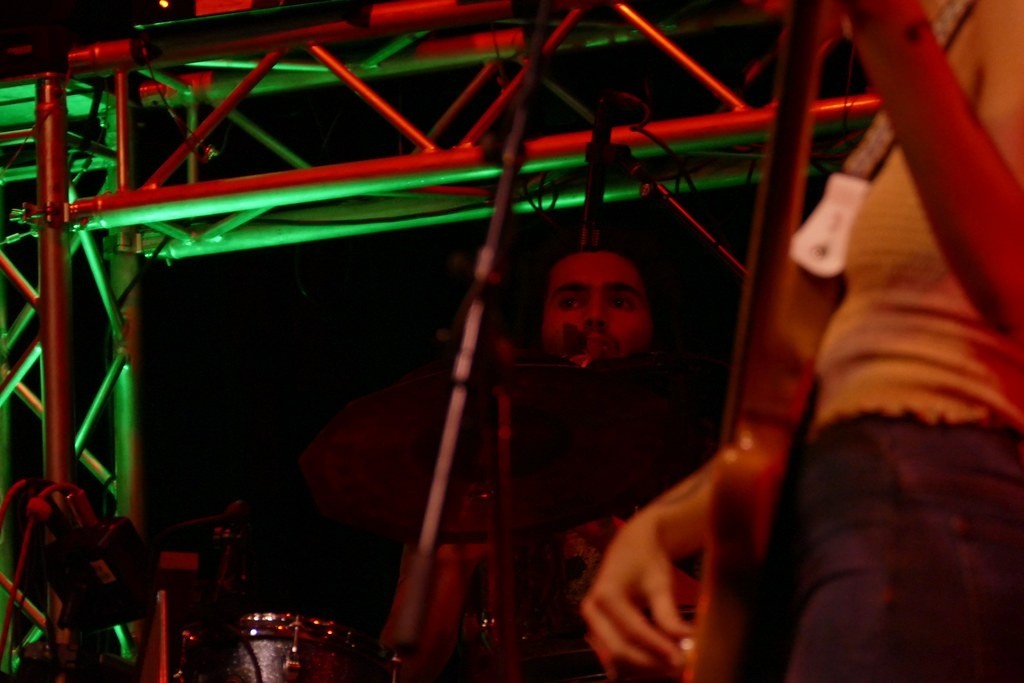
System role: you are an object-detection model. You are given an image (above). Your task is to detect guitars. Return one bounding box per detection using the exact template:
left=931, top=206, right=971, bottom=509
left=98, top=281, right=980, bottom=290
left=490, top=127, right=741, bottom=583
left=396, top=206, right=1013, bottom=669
left=684, top=0, right=839, bottom=683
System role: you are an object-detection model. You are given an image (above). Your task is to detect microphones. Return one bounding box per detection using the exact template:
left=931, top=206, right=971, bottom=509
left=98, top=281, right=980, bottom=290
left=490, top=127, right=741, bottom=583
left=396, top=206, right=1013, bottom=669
left=578, top=89, right=619, bottom=252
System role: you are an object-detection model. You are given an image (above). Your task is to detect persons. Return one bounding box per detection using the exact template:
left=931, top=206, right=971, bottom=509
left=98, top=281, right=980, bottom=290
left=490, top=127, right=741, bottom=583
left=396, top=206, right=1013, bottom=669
left=581, top=1, right=1024, bottom=682
left=300, top=247, right=726, bottom=683
left=26, top=490, right=148, bottom=677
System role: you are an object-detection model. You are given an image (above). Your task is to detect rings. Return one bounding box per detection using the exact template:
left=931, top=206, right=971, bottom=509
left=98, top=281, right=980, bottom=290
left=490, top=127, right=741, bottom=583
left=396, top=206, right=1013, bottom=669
left=477, top=480, right=498, bottom=507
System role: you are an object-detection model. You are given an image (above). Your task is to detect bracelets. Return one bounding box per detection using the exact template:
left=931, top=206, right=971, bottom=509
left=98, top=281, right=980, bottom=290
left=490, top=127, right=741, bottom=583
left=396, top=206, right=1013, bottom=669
left=745, top=411, right=792, bottom=431
left=475, top=604, right=495, bottom=651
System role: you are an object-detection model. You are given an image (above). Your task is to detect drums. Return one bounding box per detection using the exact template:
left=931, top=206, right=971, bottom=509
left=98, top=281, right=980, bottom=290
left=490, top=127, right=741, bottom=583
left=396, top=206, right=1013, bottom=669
left=231, top=609, right=356, bottom=683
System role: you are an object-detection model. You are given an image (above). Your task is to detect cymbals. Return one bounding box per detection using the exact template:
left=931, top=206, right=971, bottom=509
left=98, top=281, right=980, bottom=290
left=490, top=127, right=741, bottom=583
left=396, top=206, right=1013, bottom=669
left=299, top=361, right=691, bottom=550
left=157, top=514, right=300, bottom=585
